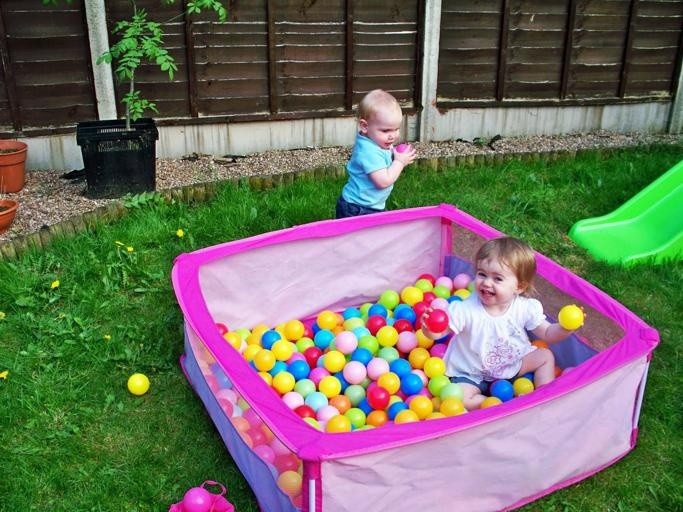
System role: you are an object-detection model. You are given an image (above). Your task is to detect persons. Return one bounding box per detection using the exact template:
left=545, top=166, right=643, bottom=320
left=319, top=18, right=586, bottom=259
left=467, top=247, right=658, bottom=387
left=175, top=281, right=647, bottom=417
left=420, top=235, right=585, bottom=411
left=333, top=86, right=416, bottom=218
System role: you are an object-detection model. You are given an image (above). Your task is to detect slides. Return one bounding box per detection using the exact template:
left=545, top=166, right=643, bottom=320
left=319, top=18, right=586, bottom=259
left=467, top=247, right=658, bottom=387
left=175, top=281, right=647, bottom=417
left=569, top=160, right=682, bottom=267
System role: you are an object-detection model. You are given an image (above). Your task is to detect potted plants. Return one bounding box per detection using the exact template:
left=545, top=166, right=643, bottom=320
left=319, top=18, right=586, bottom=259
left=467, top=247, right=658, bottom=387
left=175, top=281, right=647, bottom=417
left=42, top=0, right=227, bottom=200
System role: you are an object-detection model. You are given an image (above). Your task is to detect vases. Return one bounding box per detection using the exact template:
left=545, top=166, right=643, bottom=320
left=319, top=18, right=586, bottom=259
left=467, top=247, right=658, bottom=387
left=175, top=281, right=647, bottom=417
left=0, top=140, right=28, bottom=234
